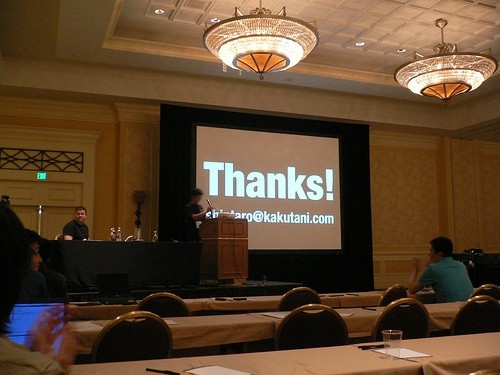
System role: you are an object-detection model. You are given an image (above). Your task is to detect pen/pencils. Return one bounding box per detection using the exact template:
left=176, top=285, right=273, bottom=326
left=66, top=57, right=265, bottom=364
left=215, top=298, right=226, bottom=301
left=146, top=368, right=180, bottom=375
left=366, top=308, right=376, bottom=311
left=358, top=344, right=390, bottom=350
left=233, top=298, right=247, bottom=300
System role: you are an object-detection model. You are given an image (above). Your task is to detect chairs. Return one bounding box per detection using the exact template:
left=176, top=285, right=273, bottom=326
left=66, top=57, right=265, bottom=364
left=471, top=284, right=500, bottom=301
left=90, top=311, right=172, bottom=363
left=137, top=292, right=191, bottom=317
left=379, top=284, right=407, bottom=306
left=369, top=298, right=431, bottom=339
left=450, top=296, right=500, bottom=335
left=278, top=288, right=322, bottom=311
left=275, top=303, right=349, bottom=350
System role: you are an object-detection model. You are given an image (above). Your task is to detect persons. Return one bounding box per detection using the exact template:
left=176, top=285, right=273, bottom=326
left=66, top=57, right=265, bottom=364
left=408, top=236, right=474, bottom=304
left=63, top=206, right=89, bottom=240
left=0, top=204, right=47, bottom=330
left=185, top=189, right=216, bottom=241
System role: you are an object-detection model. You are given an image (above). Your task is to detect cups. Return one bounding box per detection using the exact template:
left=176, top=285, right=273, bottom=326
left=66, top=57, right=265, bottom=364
left=382, top=330, right=404, bottom=360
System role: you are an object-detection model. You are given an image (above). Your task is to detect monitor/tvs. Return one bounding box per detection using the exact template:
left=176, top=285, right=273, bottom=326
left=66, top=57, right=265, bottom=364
left=8, top=298, right=69, bottom=360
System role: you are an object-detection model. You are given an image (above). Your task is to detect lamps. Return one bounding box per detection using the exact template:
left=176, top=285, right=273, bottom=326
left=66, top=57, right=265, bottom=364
left=394, top=19, right=497, bottom=102
left=202, top=0, right=319, bottom=81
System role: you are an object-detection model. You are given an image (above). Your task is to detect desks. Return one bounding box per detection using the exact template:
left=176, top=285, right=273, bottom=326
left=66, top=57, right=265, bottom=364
left=42, top=241, right=500, bottom=375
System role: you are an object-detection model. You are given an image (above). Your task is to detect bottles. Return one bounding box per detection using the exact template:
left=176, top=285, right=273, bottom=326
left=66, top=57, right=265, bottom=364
left=110, top=226, right=122, bottom=241
left=151, top=231, right=158, bottom=242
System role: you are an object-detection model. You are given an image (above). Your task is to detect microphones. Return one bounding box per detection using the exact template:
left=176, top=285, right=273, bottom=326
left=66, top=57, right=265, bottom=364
left=207, top=200, right=211, bottom=205
